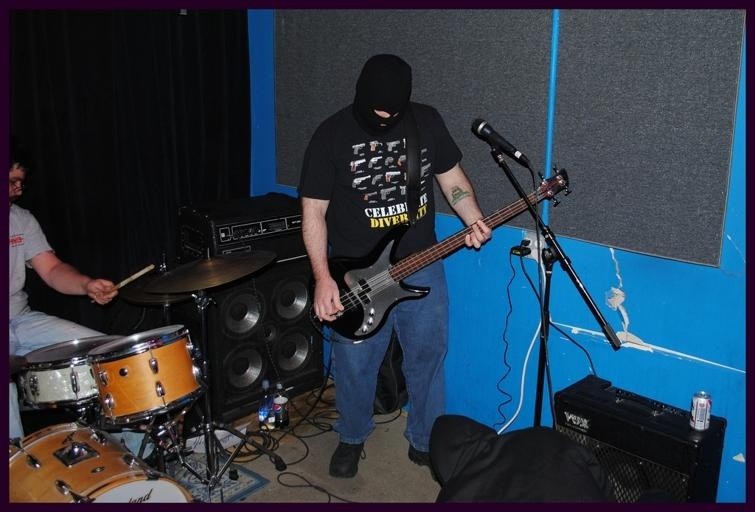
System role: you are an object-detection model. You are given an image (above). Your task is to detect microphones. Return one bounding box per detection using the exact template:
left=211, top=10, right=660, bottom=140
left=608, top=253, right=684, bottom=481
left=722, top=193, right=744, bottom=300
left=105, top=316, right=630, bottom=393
left=471, top=117, right=532, bottom=169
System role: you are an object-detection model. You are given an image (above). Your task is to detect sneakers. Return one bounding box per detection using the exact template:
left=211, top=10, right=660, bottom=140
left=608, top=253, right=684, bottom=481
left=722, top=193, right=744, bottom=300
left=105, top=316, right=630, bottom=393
left=329, top=438, right=363, bottom=479
left=408, top=443, right=443, bottom=482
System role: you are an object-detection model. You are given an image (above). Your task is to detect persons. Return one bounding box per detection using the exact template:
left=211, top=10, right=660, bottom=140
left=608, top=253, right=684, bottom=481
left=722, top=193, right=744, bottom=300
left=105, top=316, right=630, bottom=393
left=294, top=53, right=495, bottom=479
left=424, top=413, right=622, bottom=506
left=9, top=151, right=165, bottom=463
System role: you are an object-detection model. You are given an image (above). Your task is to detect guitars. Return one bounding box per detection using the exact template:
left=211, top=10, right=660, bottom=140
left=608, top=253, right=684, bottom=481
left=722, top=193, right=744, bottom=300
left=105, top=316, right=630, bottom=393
left=319, top=167, right=571, bottom=343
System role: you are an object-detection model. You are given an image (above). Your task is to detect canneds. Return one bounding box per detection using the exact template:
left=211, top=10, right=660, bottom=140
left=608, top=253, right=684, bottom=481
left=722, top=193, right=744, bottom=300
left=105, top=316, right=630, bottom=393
left=689, top=391, right=712, bottom=432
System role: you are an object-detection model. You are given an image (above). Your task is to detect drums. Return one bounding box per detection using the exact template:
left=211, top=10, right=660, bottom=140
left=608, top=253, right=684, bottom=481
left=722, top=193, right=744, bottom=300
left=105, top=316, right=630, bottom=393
left=21, top=334, right=125, bottom=410
left=86, top=325, right=207, bottom=424
left=8, top=422, right=192, bottom=503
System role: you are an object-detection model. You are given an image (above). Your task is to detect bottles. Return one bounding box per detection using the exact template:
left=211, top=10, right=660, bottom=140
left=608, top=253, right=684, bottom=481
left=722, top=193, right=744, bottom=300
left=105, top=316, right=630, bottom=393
left=257, top=378, right=290, bottom=430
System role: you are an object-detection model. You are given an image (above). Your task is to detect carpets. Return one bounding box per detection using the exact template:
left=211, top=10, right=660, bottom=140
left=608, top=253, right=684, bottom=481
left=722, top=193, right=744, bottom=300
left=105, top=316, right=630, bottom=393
left=164, top=453, right=271, bottom=503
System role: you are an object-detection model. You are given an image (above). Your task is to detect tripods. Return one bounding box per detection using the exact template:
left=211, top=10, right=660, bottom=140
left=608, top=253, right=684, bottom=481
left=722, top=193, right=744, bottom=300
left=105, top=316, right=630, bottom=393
left=169, top=300, right=288, bottom=492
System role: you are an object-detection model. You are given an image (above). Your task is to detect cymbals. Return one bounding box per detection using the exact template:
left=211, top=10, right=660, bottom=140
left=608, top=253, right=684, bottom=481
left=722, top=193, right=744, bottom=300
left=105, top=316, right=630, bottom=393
left=121, top=279, right=198, bottom=304
left=143, top=251, right=277, bottom=294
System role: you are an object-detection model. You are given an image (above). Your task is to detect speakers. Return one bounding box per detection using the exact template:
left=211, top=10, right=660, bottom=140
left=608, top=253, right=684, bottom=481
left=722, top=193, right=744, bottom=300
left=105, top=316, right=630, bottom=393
left=172, top=233, right=325, bottom=426
left=553, top=375, right=728, bottom=503
